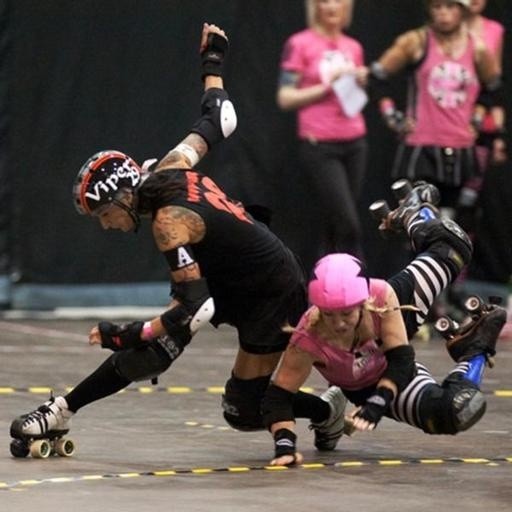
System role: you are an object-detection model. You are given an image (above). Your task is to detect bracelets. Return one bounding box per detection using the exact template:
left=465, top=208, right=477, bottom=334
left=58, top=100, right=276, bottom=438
left=143, top=320, right=155, bottom=339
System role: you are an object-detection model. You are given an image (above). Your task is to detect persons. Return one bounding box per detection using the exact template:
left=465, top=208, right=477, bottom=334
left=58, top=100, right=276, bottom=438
left=357, top=0, right=502, bottom=341
left=9, top=22, right=348, bottom=451
left=452, top=0, right=511, bottom=287
left=276, top=0, right=370, bottom=281
left=260, top=184, right=506, bottom=467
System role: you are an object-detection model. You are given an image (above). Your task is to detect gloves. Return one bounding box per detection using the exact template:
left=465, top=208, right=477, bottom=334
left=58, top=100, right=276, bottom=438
left=273, top=428, right=297, bottom=466
left=352, top=385, right=395, bottom=430
left=387, top=109, right=411, bottom=133
left=97, top=319, right=146, bottom=352
left=199, top=31, right=230, bottom=83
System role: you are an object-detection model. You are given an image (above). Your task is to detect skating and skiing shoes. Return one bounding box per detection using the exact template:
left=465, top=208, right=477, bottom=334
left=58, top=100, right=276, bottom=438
left=9, top=390, right=76, bottom=460
left=433, top=294, right=508, bottom=369
left=308, top=385, right=357, bottom=451
left=368, top=178, right=441, bottom=243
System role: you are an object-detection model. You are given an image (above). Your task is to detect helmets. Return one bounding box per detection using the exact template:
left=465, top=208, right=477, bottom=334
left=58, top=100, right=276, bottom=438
left=307, top=253, right=370, bottom=312
left=72, top=150, right=144, bottom=218
